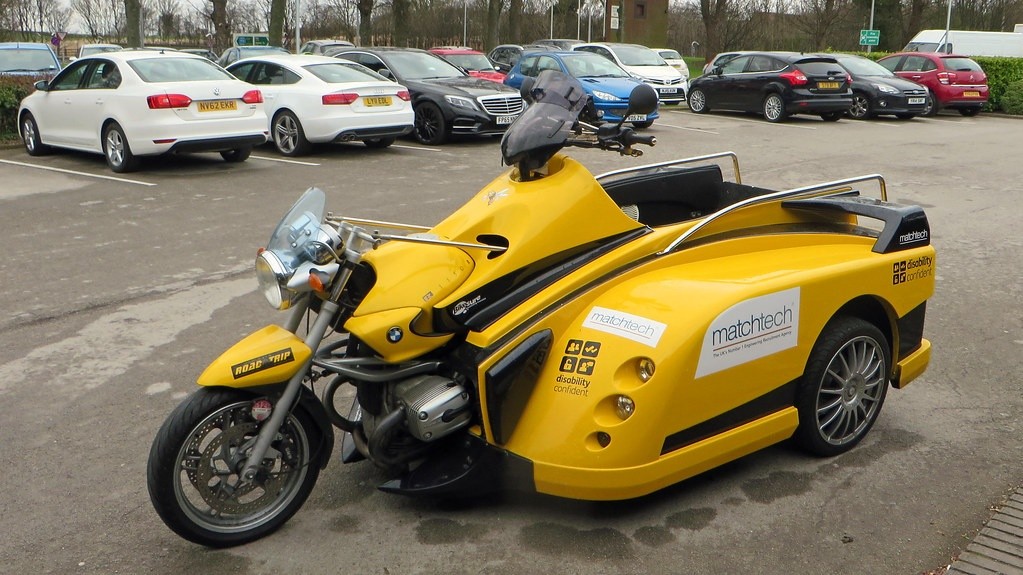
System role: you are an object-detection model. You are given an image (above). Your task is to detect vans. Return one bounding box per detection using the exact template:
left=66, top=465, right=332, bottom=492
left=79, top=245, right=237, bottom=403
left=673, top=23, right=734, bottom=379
left=900, top=29, right=1023, bottom=72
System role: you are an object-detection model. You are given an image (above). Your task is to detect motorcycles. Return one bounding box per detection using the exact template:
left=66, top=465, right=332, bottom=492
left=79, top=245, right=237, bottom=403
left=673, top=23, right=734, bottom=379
left=145, top=69, right=935, bottom=548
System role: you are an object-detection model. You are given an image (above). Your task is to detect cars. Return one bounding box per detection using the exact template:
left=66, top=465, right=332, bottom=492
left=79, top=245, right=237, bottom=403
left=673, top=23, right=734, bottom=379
left=0, top=40, right=356, bottom=76
left=223, top=46, right=415, bottom=157
left=503, top=50, right=660, bottom=129
left=874, top=52, right=989, bottom=117
left=687, top=51, right=930, bottom=123
left=299, top=39, right=524, bottom=145
left=487, top=38, right=690, bottom=105
left=17, top=50, right=270, bottom=173
